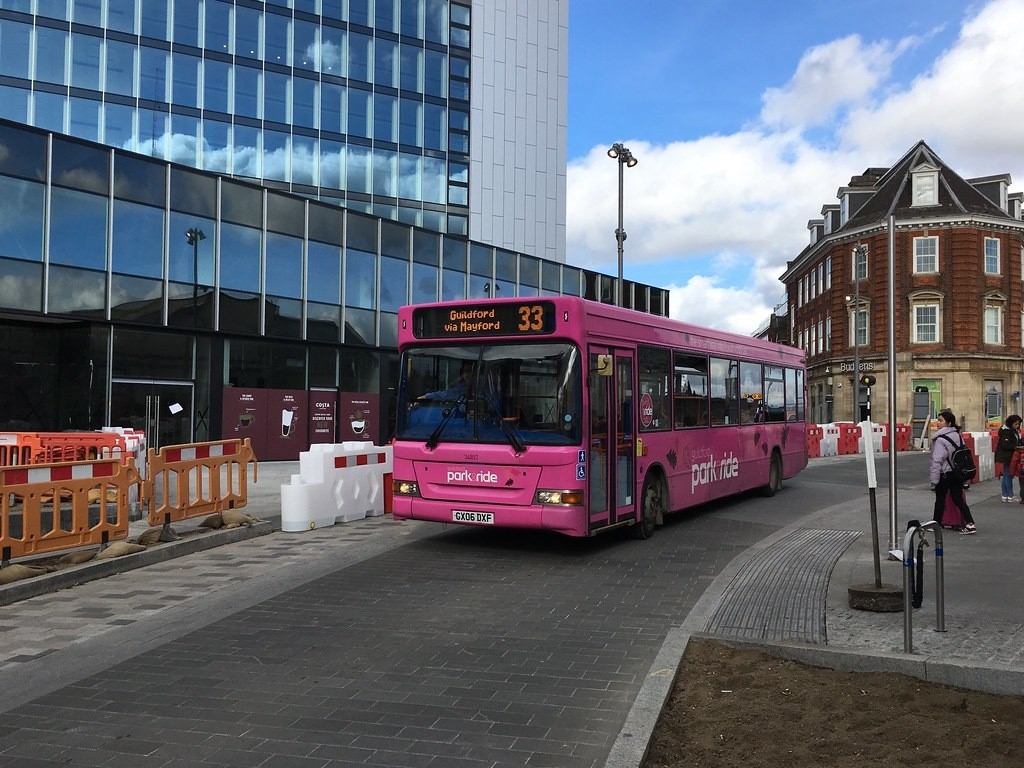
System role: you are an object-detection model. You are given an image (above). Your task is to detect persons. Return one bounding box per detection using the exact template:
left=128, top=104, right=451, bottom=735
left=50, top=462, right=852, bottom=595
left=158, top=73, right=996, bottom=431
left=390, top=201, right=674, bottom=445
left=410, top=361, right=501, bottom=427
left=993, top=414, right=1024, bottom=504
left=925, top=412, right=976, bottom=535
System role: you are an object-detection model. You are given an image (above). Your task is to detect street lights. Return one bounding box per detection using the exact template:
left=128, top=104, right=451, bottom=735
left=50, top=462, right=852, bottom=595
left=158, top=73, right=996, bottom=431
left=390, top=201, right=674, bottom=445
left=607, top=142, right=637, bottom=306
left=851, top=244, right=871, bottom=421
left=186, top=226, right=206, bottom=326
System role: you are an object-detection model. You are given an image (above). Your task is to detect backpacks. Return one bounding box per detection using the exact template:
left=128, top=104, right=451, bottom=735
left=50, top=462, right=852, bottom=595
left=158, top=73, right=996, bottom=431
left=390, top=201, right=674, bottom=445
left=938, top=434, right=976, bottom=482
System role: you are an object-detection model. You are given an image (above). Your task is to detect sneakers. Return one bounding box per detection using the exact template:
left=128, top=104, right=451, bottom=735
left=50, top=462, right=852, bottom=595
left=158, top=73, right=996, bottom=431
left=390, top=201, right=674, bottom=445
left=1002, top=496, right=1009, bottom=503
left=1007, top=496, right=1022, bottom=503
left=959, top=525, right=977, bottom=534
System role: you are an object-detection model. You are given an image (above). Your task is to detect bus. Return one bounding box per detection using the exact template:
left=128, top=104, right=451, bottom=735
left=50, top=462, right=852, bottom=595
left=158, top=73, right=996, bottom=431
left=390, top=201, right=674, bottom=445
left=392, top=296, right=809, bottom=540
